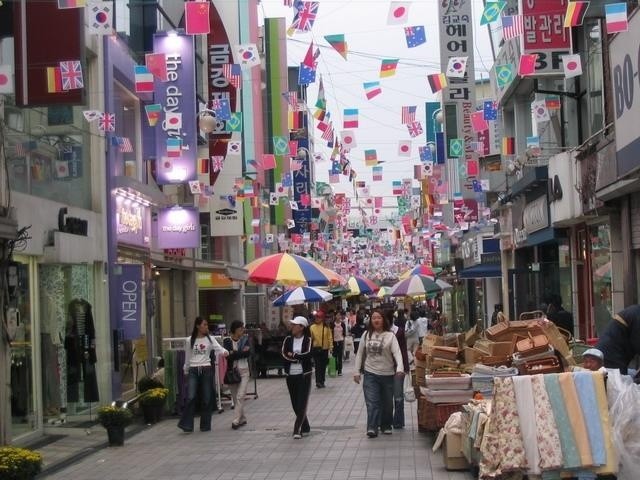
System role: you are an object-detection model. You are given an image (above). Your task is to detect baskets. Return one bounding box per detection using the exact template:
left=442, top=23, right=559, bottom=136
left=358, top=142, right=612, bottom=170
left=413, top=311, right=561, bottom=433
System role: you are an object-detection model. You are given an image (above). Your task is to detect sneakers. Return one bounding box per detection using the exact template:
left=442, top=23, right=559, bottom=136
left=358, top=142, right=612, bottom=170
left=240, top=419, right=247, bottom=425
left=408, top=360, right=414, bottom=365
left=293, top=434, right=301, bottom=439
left=301, top=432, right=310, bottom=436
left=316, top=382, right=323, bottom=388
left=367, top=424, right=402, bottom=438
left=338, top=370, right=342, bottom=376
left=232, top=424, right=239, bottom=429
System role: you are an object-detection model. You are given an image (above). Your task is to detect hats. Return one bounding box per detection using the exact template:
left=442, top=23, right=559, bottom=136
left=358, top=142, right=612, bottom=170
left=582, top=349, right=604, bottom=360
left=289, top=316, right=308, bottom=327
left=316, top=311, right=325, bottom=318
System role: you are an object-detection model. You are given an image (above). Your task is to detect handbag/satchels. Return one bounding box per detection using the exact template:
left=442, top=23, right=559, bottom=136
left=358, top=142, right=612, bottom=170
left=312, top=347, right=322, bottom=355
left=403, top=373, right=416, bottom=402
left=328, top=357, right=336, bottom=377
left=361, top=346, right=366, bottom=374
left=223, top=367, right=241, bottom=384
left=406, top=324, right=416, bottom=338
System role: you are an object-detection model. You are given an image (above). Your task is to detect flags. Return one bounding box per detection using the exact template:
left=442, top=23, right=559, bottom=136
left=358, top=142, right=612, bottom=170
left=1, top=0, right=631, bottom=279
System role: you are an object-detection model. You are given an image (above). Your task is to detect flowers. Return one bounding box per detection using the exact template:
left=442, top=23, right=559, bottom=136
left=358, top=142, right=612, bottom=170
left=0, top=444, right=44, bottom=479
left=137, top=388, right=170, bottom=401
left=96, top=405, right=135, bottom=429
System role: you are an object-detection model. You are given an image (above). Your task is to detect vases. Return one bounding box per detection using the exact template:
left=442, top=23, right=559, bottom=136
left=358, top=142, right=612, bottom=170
left=144, top=402, right=161, bottom=424
left=106, top=427, right=124, bottom=446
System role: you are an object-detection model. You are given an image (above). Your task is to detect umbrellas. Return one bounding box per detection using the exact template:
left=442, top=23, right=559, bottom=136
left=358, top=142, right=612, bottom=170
left=240, top=251, right=455, bottom=308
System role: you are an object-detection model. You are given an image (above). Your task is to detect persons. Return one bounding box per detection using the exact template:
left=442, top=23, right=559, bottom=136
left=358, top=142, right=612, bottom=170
left=281, top=315, right=314, bottom=439
left=212, top=307, right=441, bottom=389
left=176, top=315, right=231, bottom=433
left=546, top=294, right=575, bottom=338
left=581, top=347, right=605, bottom=371
left=352, top=309, right=405, bottom=439
left=593, top=301, right=640, bottom=375
left=145, top=358, right=167, bottom=390
left=376, top=309, right=411, bottom=431
left=222, top=320, right=256, bottom=430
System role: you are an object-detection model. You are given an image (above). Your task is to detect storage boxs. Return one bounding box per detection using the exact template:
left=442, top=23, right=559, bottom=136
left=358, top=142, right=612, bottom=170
left=431, top=428, right=472, bottom=471
